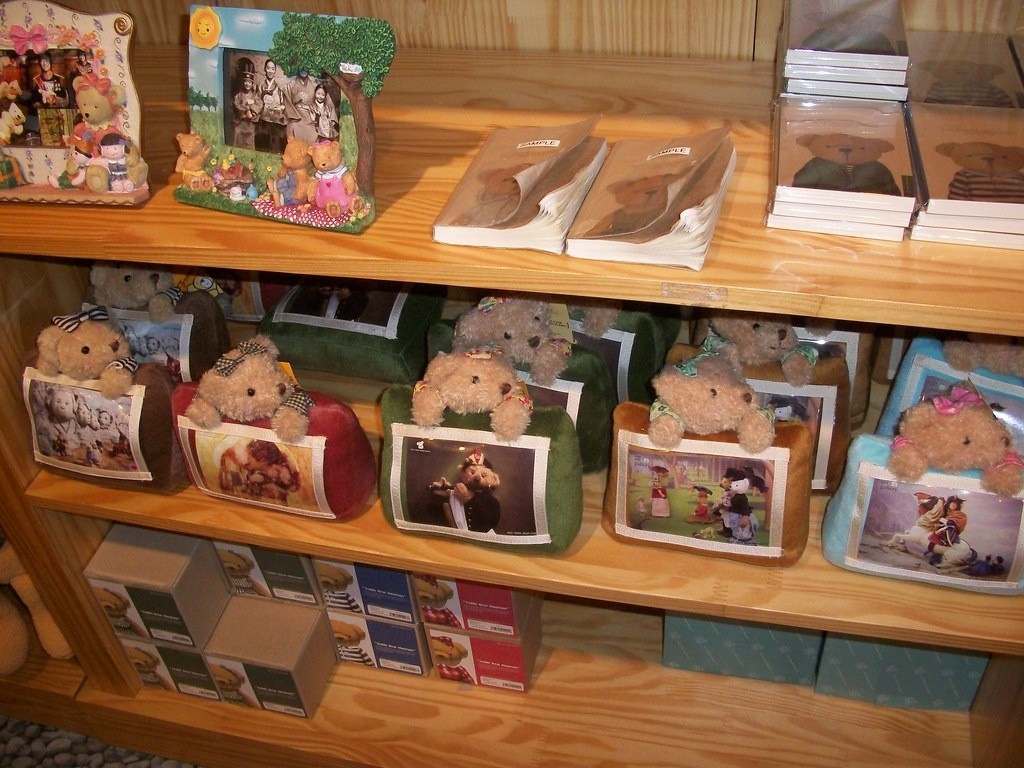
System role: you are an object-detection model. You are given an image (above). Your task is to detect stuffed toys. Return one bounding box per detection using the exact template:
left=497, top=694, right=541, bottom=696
left=66, top=72, right=148, bottom=193
left=429, top=450, right=500, bottom=536
left=32, top=263, right=1024, bottom=494
left=173, top=131, right=363, bottom=217
left=0, top=526, right=76, bottom=676
left=91, top=549, right=475, bottom=713
left=0, top=55, right=26, bottom=148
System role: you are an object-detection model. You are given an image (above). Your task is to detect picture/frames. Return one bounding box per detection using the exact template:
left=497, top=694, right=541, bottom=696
left=0, top=0, right=150, bottom=206
left=172, top=6, right=396, bottom=235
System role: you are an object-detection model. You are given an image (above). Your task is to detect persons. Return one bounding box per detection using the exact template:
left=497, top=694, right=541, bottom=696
left=46, top=131, right=136, bottom=192
left=229, top=58, right=340, bottom=157
left=30, top=51, right=93, bottom=106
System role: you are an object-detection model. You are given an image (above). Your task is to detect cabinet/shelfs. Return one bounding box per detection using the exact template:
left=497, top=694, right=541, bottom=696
left=0, top=46, right=1024, bottom=768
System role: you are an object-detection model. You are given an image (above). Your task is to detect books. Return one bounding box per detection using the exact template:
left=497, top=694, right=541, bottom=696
left=432, top=0, right=1024, bottom=271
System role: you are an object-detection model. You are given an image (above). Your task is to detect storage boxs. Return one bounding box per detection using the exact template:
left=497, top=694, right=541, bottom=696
left=662, top=610, right=987, bottom=712
left=81, top=522, right=541, bottom=719
